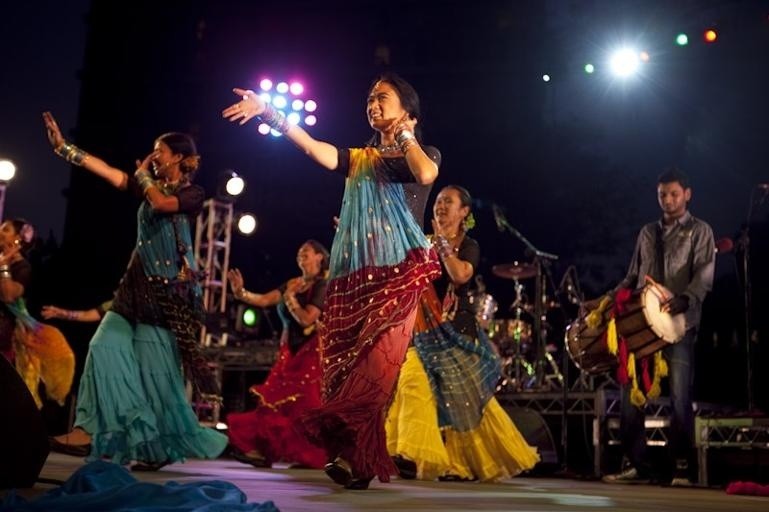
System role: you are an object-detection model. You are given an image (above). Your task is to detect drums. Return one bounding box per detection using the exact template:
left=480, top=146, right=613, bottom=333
left=458, top=289, right=498, bottom=333
left=488, top=308, right=535, bottom=355
left=564, top=283, right=686, bottom=376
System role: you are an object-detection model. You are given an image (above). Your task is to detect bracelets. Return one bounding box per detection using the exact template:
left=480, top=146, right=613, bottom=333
left=66, top=310, right=79, bottom=322
left=433, top=234, right=458, bottom=263
left=392, top=124, right=418, bottom=152
left=0, top=264, right=13, bottom=279
left=284, top=297, right=300, bottom=312
left=54, top=140, right=89, bottom=166
left=235, top=288, right=253, bottom=302
left=260, top=105, right=291, bottom=134
left=133, top=168, right=157, bottom=196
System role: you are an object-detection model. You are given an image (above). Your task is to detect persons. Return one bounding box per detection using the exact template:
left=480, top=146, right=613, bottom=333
left=40, top=109, right=235, bottom=477
left=222, top=236, right=340, bottom=469
left=574, top=164, right=720, bottom=487
left=330, top=183, right=544, bottom=486
left=0, top=216, right=80, bottom=414
left=220, top=73, right=446, bottom=492
left=36, top=294, right=120, bottom=324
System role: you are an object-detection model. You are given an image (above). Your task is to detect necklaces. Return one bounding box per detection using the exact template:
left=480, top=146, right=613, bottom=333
left=375, top=142, right=402, bottom=154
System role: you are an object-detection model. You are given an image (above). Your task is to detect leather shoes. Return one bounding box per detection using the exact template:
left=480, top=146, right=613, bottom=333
left=391, top=456, right=416, bottom=479
left=46, top=436, right=93, bottom=458
left=325, top=462, right=368, bottom=490
left=438, top=474, right=478, bottom=483
left=131, top=457, right=170, bottom=472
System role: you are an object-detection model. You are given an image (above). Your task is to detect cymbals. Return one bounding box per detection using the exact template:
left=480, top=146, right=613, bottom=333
left=490, top=263, right=538, bottom=280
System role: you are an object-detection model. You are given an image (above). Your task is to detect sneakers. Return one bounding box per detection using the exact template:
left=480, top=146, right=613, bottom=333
left=669, top=471, right=694, bottom=486
left=603, top=467, right=648, bottom=483
